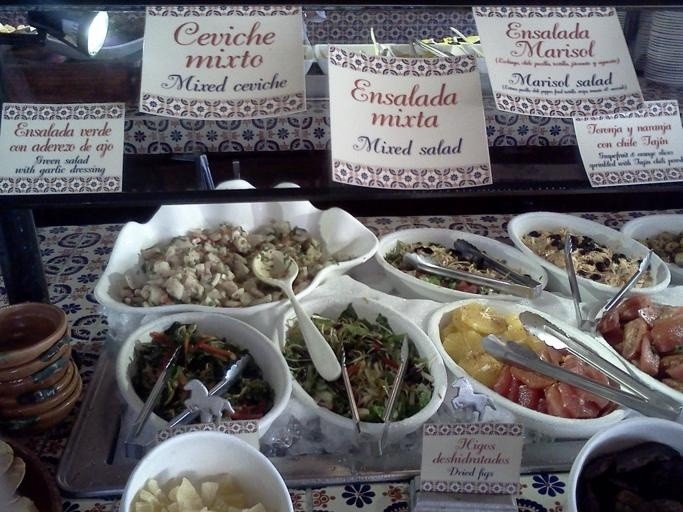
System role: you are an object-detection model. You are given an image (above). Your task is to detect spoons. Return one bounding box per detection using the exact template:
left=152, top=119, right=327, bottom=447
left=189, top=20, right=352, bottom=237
left=250, top=247, right=342, bottom=381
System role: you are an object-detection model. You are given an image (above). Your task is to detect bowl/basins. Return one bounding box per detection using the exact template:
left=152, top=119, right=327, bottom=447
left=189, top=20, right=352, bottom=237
left=564, top=418, right=683, bottom=512
left=430, top=290, right=635, bottom=430
left=117, top=432, right=292, bottom=512
left=0, top=302, right=83, bottom=436
left=112, top=312, right=294, bottom=441
left=274, top=294, right=448, bottom=445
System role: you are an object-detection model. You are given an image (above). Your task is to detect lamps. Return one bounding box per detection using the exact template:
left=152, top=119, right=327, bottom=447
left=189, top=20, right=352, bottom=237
left=20, top=8, right=114, bottom=60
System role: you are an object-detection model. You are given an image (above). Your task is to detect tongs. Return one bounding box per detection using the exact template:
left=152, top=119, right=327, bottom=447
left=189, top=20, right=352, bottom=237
left=402, top=237, right=546, bottom=298
left=562, top=231, right=655, bottom=332
left=482, top=310, right=682, bottom=424
left=124, top=320, right=252, bottom=463
left=333, top=332, right=410, bottom=457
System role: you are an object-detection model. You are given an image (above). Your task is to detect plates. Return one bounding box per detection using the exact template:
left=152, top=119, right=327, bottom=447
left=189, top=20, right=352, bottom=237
left=505, top=211, right=674, bottom=298
left=603, top=291, right=683, bottom=405
left=377, top=225, right=549, bottom=298
left=98, top=197, right=383, bottom=313
